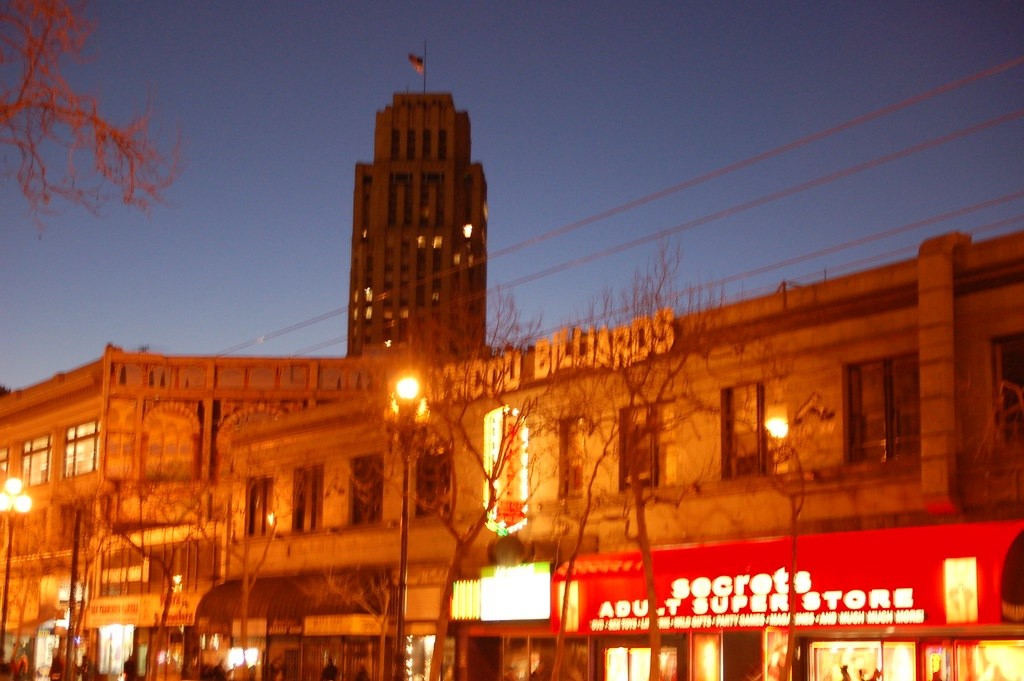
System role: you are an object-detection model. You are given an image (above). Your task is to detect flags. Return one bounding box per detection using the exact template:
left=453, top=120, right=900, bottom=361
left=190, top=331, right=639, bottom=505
left=407, top=52, right=424, bottom=74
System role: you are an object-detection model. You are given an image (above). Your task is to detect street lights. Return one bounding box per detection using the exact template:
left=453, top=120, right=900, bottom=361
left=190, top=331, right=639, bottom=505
left=385, top=367, right=432, bottom=681
left=1, top=477, right=33, bottom=665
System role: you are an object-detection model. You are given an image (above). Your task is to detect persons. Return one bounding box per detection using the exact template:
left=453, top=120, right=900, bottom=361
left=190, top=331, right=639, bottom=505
left=838, top=665, right=851, bottom=681
left=320, top=656, right=337, bottom=681
left=47, top=647, right=63, bottom=681
left=858, top=668, right=866, bottom=681
left=123, top=653, right=136, bottom=681
left=202, top=659, right=237, bottom=681
left=79, top=656, right=91, bottom=681
